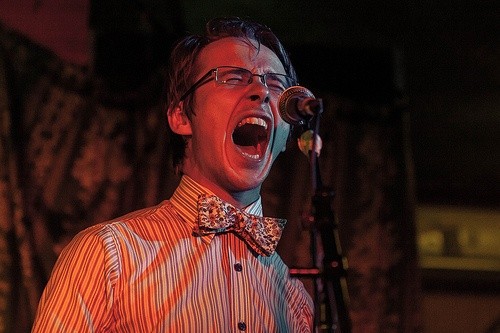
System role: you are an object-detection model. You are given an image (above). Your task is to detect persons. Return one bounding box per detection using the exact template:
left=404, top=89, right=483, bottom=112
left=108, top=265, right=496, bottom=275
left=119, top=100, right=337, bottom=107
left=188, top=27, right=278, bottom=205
left=31, top=16, right=315, bottom=333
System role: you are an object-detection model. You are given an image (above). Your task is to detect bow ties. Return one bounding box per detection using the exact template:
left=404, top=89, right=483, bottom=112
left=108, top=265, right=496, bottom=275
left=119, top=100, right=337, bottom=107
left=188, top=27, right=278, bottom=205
left=193, top=193, right=287, bottom=255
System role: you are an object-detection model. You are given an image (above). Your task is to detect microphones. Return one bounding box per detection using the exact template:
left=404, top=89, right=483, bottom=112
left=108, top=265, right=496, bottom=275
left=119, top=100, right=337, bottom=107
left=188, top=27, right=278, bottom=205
left=277, top=86, right=323, bottom=126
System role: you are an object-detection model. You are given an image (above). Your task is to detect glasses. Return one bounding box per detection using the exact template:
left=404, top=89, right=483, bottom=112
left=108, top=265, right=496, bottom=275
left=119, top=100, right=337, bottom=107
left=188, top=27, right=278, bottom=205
left=180, top=65, right=299, bottom=102
